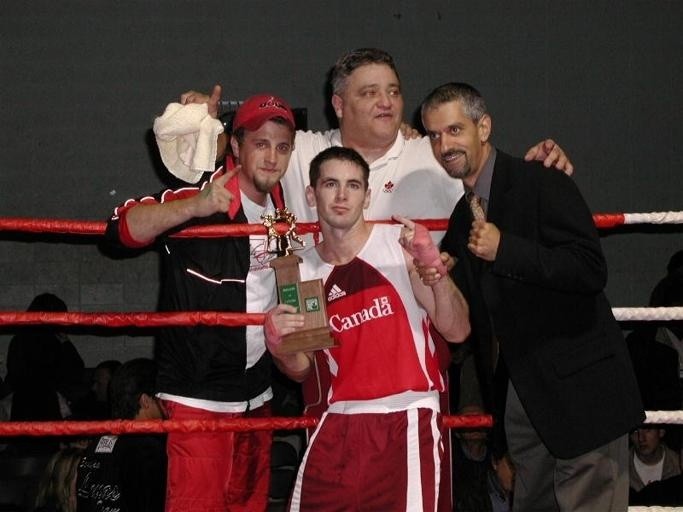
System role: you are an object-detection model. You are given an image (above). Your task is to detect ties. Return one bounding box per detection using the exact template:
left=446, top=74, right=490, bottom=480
left=467, top=192, right=499, bottom=375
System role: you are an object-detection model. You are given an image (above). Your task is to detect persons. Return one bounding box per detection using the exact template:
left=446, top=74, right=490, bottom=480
left=97, top=94, right=296, bottom=508
left=264, top=146, right=472, bottom=508
left=179, top=48, right=574, bottom=508
left=411, top=82, right=646, bottom=507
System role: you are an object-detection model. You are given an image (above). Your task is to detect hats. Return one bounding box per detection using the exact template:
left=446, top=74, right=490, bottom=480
left=231, top=94, right=295, bottom=133
